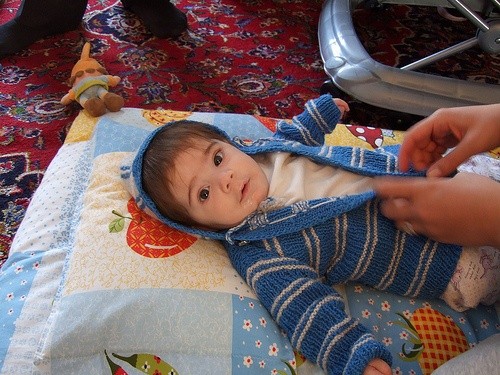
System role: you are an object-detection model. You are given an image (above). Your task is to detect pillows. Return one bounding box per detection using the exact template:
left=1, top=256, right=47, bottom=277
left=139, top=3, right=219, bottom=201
left=33, top=117, right=297, bottom=375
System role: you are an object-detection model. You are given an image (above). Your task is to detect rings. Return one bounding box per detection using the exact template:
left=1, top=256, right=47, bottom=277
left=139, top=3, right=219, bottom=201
left=402, top=221, right=416, bottom=235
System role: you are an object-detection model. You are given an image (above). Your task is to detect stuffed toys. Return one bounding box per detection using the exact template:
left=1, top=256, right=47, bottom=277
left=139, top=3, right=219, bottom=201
left=60, top=42, right=124, bottom=118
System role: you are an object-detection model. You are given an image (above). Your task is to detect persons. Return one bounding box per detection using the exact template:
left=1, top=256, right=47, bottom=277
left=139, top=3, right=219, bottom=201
left=132, top=94, right=500, bottom=375
left=0, top=0, right=188, bottom=57
left=373, top=103, right=500, bottom=247
